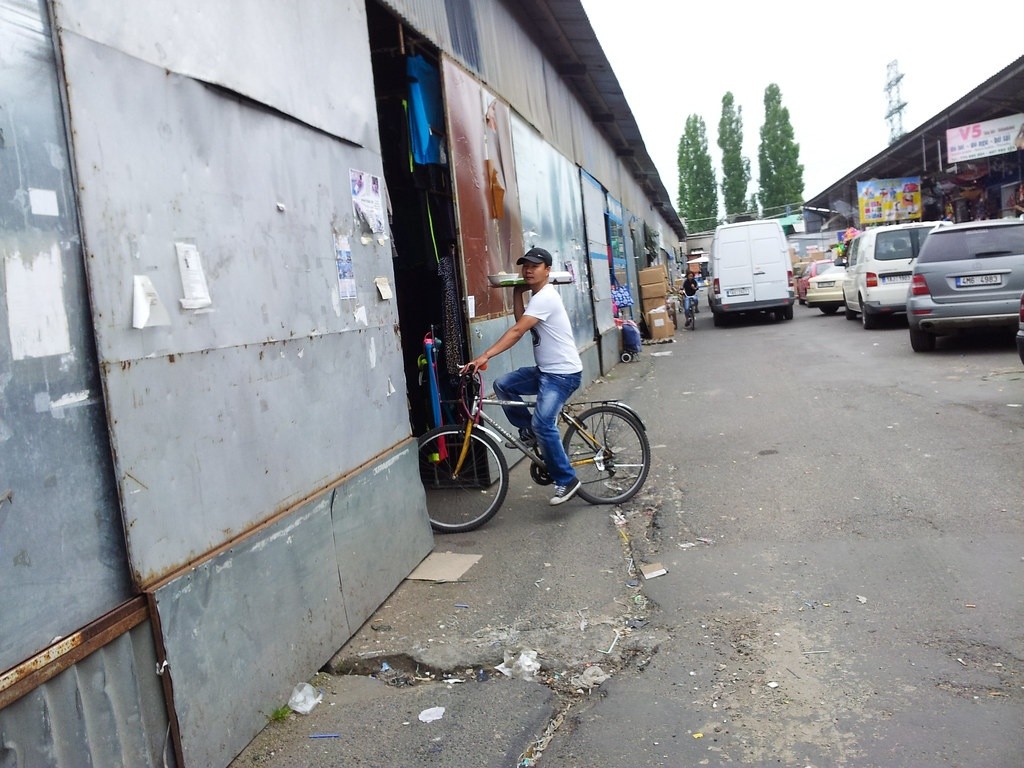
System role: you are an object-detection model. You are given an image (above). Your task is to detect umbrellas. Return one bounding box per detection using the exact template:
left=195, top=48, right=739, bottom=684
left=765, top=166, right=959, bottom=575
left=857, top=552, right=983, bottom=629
left=686, top=257, right=709, bottom=273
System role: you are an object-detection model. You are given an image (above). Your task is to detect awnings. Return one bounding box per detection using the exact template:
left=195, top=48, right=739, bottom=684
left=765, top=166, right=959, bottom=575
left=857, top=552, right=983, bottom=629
left=819, top=214, right=854, bottom=231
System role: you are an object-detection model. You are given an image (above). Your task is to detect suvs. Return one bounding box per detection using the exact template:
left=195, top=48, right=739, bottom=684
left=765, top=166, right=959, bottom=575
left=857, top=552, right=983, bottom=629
left=905, top=216, right=1024, bottom=352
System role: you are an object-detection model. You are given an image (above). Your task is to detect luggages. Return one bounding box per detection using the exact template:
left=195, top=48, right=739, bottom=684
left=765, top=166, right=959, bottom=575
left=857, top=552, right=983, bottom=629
left=617, top=306, right=642, bottom=363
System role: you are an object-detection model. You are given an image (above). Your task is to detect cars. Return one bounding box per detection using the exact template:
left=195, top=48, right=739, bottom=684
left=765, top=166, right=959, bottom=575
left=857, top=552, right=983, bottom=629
left=796, top=258, right=835, bottom=305
left=806, top=257, right=847, bottom=314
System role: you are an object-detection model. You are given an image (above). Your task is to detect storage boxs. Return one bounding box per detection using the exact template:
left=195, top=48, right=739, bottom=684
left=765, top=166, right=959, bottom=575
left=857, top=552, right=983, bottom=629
left=639, top=264, right=675, bottom=339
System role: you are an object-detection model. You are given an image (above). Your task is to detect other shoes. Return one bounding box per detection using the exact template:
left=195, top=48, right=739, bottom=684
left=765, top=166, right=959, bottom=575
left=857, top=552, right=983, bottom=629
left=695, top=309, right=699, bottom=313
left=684, top=320, right=690, bottom=326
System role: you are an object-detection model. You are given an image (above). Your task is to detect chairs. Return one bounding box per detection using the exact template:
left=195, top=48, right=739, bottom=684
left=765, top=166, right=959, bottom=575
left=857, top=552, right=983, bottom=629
left=893, top=238, right=907, bottom=253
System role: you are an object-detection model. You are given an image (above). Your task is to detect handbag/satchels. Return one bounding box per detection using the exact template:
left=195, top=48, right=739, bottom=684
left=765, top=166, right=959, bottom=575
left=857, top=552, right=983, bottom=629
left=611, top=275, right=635, bottom=307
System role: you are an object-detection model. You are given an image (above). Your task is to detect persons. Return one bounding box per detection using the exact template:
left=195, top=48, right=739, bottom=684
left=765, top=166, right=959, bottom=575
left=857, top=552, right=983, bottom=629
left=697, top=268, right=710, bottom=277
left=457, top=247, right=583, bottom=505
left=678, top=271, right=700, bottom=327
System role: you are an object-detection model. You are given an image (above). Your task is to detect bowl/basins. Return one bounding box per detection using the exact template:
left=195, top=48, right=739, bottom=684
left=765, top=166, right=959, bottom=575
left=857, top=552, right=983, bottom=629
left=486, top=273, right=519, bottom=284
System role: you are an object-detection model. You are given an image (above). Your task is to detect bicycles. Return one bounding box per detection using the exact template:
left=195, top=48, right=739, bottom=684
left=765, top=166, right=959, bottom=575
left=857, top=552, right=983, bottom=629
left=417, top=364, right=652, bottom=535
left=682, top=287, right=701, bottom=331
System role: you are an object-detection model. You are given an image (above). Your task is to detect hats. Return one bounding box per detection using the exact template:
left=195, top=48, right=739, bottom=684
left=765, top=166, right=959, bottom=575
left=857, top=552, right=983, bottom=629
left=516, top=247, right=552, bottom=267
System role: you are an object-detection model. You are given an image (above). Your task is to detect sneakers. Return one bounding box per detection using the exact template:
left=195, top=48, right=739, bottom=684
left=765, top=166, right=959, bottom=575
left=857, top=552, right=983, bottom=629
left=549, top=476, right=581, bottom=505
left=505, top=430, right=538, bottom=449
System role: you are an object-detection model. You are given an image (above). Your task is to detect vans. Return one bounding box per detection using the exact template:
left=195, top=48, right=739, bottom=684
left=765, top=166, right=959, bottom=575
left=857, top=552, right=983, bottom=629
left=842, top=219, right=953, bottom=329
left=706, top=219, right=797, bottom=327
